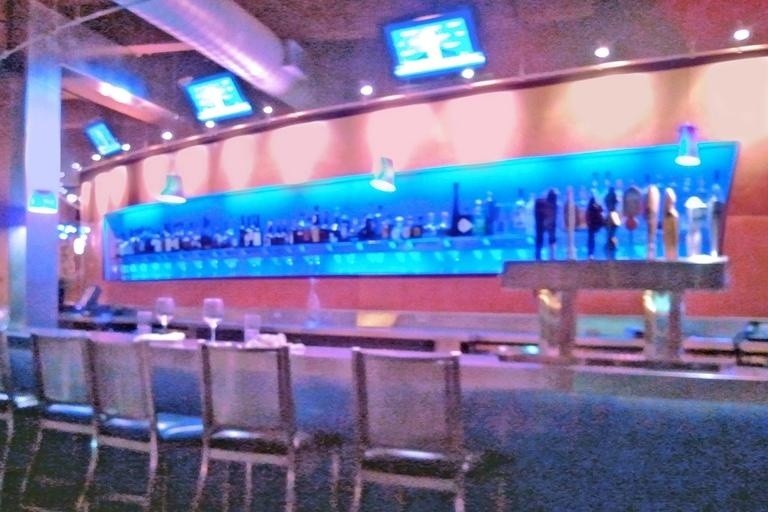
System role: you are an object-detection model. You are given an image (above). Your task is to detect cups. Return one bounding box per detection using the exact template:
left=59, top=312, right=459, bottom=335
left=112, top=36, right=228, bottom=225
left=137, top=310, right=153, bottom=334
left=244, top=314, right=260, bottom=340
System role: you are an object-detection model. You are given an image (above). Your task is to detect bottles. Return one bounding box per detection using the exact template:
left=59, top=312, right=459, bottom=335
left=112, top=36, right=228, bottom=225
left=115, top=168, right=721, bottom=258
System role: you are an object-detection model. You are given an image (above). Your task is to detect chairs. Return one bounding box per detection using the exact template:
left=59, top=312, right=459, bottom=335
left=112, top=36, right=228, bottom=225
left=0, top=387, right=37, bottom=496
left=20, top=330, right=108, bottom=497
left=77, top=336, right=205, bottom=510
left=191, top=339, right=354, bottom=510
left=351, top=344, right=484, bottom=512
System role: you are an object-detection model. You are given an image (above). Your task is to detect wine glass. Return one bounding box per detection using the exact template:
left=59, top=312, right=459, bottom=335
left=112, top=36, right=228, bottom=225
left=155, top=296, right=176, bottom=333
left=201, top=298, right=225, bottom=343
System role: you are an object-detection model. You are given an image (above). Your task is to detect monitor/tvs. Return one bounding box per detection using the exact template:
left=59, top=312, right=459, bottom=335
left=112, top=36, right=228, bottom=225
left=82, top=117, right=123, bottom=159
left=382, top=6, right=487, bottom=81
left=180, top=71, right=256, bottom=126
